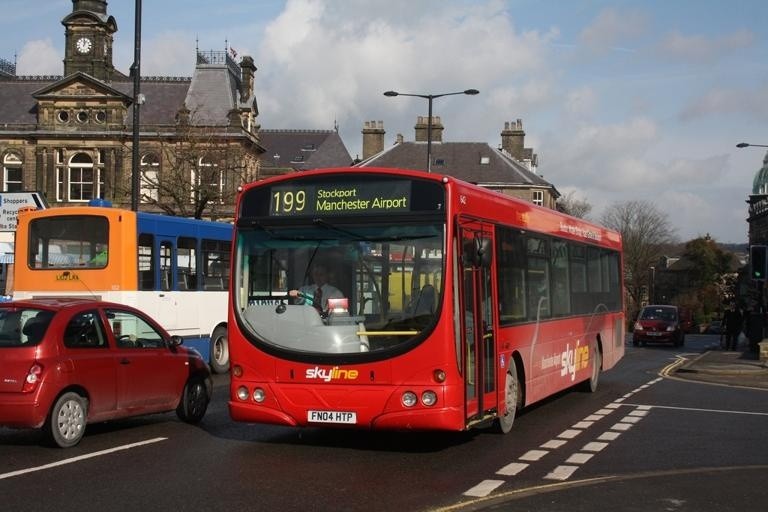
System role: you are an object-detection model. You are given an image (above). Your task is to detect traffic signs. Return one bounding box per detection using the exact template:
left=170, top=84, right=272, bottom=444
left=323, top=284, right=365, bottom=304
left=0, top=191, right=51, bottom=234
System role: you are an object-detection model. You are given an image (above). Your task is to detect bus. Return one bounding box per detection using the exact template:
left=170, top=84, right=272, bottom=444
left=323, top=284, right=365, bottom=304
left=7, top=197, right=236, bottom=376
left=218, top=165, right=631, bottom=448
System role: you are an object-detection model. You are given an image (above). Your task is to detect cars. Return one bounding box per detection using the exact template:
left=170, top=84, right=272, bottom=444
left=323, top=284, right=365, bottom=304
left=1, top=296, right=219, bottom=450
left=630, top=303, right=691, bottom=349
left=709, top=320, right=722, bottom=334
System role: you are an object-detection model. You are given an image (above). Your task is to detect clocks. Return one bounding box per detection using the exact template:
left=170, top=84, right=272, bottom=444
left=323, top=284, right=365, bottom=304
left=76, top=37, right=92, bottom=53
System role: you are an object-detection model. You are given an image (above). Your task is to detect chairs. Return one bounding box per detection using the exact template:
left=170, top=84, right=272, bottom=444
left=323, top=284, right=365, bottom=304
left=156, top=269, right=229, bottom=291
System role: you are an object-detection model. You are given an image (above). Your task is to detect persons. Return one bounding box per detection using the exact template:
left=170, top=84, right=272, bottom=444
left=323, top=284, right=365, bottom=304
left=289, top=264, right=344, bottom=320
left=720, top=300, right=765, bottom=351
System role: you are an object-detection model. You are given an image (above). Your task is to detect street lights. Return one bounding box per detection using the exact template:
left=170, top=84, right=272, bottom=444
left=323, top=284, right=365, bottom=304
left=381, top=87, right=482, bottom=174
left=734, top=143, right=768, bottom=149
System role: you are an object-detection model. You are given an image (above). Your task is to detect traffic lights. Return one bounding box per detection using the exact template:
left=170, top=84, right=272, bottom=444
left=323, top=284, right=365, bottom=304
left=749, top=244, right=767, bottom=282
